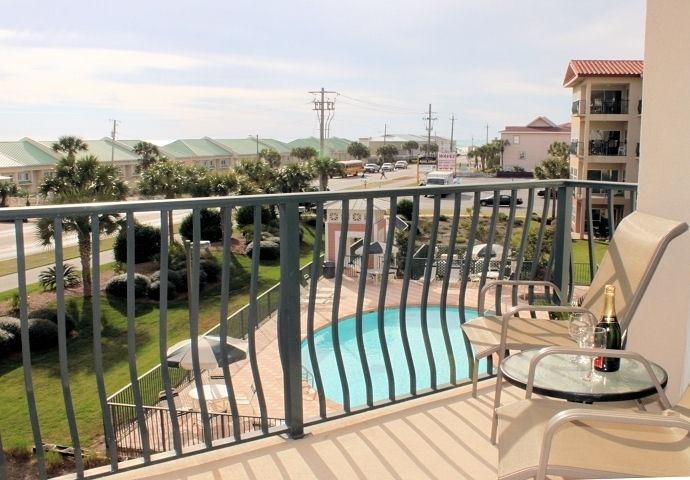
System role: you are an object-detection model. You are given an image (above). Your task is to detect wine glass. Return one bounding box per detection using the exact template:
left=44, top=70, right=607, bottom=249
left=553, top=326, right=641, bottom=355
left=567, top=312, right=593, bottom=365
left=577, top=325, right=609, bottom=385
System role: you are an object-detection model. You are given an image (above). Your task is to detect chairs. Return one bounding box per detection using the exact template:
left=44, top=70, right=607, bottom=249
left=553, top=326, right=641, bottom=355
left=460, top=209, right=689, bottom=446
left=494, top=384, right=690, bottom=480
left=355, top=268, right=397, bottom=288
left=158, top=372, right=256, bottom=442
left=470, top=268, right=513, bottom=289
left=448, top=264, right=460, bottom=288
left=418, top=263, right=438, bottom=285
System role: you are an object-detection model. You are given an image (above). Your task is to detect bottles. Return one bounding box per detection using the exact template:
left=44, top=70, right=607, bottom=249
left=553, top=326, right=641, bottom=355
left=591, top=284, right=623, bottom=372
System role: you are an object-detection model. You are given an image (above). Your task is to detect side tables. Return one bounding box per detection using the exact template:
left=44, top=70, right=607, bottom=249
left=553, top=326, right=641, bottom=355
left=498, top=347, right=667, bottom=447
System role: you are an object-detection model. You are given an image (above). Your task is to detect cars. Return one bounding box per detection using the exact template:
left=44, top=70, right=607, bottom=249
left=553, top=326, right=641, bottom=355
left=480, top=195, right=523, bottom=207
left=537, top=188, right=559, bottom=198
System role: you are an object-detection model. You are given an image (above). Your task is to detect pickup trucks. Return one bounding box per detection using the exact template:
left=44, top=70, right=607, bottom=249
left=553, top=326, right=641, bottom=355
left=395, top=160, right=409, bottom=170
left=365, top=164, right=381, bottom=173
left=381, top=163, right=395, bottom=172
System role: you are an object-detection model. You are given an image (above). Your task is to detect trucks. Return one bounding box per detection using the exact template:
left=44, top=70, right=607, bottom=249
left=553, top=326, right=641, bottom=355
left=426, top=171, right=455, bottom=198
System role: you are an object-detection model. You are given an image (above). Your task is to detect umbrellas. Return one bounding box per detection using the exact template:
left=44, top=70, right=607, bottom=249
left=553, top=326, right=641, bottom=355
left=462, top=242, right=513, bottom=272
left=166, top=333, right=248, bottom=394
left=354, top=240, right=400, bottom=270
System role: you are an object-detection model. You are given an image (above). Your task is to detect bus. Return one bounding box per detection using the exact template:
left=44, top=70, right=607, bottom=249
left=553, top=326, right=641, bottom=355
left=329, top=160, right=366, bottom=178
